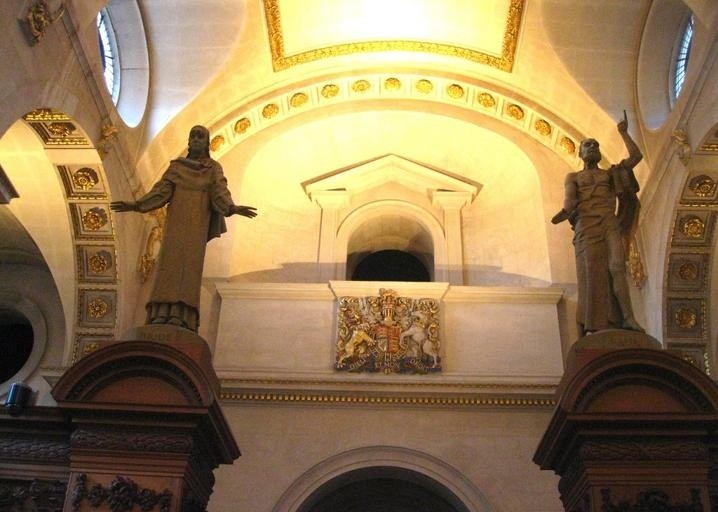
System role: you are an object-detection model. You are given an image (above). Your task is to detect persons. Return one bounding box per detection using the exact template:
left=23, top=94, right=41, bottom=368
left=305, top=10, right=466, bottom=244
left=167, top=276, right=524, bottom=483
left=111, top=121, right=258, bottom=326
left=550, top=103, right=648, bottom=329
